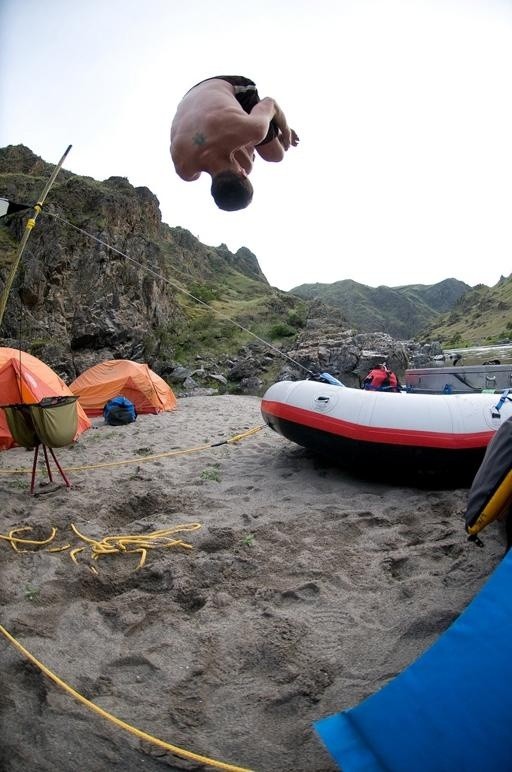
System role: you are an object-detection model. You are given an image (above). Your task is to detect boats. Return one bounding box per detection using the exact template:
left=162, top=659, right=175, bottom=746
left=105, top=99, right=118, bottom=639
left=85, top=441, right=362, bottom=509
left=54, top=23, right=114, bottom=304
left=261, top=364, right=512, bottom=485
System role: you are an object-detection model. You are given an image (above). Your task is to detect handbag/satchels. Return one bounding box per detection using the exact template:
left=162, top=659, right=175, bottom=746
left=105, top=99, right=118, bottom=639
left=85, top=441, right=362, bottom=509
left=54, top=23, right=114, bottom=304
left=104, top=397, right=137, bottom=425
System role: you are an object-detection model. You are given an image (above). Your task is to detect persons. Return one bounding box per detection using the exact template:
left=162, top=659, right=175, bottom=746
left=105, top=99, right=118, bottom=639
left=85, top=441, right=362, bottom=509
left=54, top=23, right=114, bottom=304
left=169, top=75, right=301, bottom=212
left=362, top=362, right=403, bottom=393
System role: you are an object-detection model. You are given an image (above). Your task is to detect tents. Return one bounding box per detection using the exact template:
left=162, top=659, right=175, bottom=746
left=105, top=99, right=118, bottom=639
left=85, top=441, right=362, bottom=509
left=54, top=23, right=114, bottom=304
left=67, top=358, right=179, bottom=417
left=0, top=347, right=94, bottom=450
